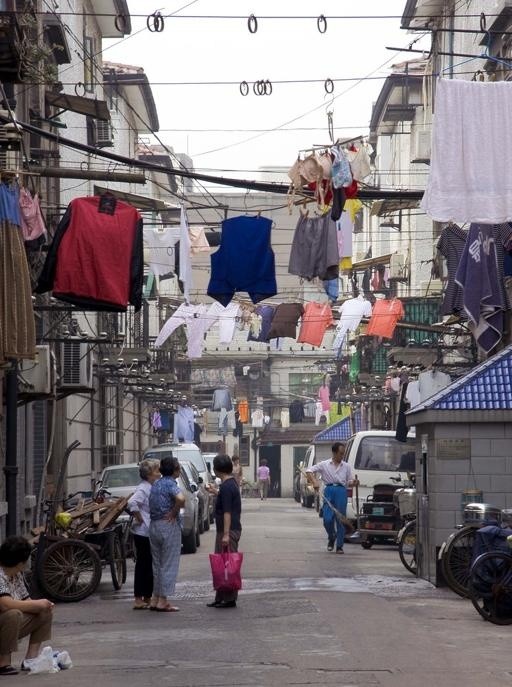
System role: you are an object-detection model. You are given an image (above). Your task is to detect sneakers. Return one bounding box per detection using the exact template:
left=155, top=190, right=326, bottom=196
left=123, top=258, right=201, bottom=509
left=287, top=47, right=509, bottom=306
left=0, top=665, right=18, bottom=675
left=328, top=539, right=335, bottom=551
left=336, top=547, right=344, bottom=554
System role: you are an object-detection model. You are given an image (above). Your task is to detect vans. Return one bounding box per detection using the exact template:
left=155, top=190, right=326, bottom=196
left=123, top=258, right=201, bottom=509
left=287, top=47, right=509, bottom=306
left=342, top=429, right=417, bottom=529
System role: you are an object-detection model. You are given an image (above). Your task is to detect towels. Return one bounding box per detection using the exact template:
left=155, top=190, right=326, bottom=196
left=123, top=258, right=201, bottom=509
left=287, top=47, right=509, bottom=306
left=420, top=76, right=512, bottom=224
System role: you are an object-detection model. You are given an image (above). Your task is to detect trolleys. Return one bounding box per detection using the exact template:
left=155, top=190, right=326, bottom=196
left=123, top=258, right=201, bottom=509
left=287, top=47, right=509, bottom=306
left=30, top=436, right=84, bottom=572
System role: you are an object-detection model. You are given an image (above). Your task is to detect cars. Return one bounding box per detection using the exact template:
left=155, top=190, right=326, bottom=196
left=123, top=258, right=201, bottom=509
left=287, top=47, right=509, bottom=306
left=292, top=442, right=328, bottom=516
left=95, top=437, right=223, bottom=555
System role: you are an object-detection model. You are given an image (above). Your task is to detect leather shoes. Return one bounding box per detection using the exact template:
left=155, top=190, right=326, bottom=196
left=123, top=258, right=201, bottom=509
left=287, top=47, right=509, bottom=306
left=132, top=600, right=179, bottom=612
left=207, top=600, right=236, bottom=607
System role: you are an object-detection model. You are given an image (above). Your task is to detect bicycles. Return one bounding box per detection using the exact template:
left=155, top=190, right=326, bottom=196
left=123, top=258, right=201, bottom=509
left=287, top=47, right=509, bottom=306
left=434, top=502, right=512, bottom=626
left=395, top=511, right=417, bottom=575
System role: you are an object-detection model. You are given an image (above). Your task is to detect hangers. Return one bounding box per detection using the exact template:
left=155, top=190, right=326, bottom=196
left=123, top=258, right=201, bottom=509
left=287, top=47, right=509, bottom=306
left=241, top=192, right=257, bottom=217
left=92, top=172, right=117, bottom=202
left=439, top=30, right=511, bottom=79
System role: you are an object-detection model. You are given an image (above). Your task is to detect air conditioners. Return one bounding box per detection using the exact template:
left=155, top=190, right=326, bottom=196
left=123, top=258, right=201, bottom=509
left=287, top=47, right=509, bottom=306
left=0, top=110, right=19, bottom=178
left=58, top=335, right=94, bottom=388
left=19, top=345, right=57, bottom=394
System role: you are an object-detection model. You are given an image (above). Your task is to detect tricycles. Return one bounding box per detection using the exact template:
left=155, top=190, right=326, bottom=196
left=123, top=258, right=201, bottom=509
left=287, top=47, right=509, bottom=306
left=356, top=474, right=416, bottom=552
left=34, top=480, right=130, bottom=602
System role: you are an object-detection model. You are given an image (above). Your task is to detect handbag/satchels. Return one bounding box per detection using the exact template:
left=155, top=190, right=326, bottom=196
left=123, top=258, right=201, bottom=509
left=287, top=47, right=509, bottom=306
left=209, top=545, right=243, bottom=591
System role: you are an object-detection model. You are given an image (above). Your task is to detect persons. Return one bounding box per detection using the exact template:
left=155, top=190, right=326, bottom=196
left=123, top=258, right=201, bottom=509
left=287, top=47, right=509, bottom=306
left=127, top=459, right=161, bottom=610
left=232, top=455, right=242, bottom=495
left=306, top=442, right=359, bottom=555
left=204, top=454, right=241, bottom=608
left=1, top=537, right=54, bottom=674
left=258, top=459, right=270, bottom=500
left=148, top=457, right=185, bottom=611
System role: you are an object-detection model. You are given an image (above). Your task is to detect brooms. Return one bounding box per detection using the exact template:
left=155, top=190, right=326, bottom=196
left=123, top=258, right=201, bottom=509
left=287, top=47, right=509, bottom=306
left=295, top=465, right=356, bottom=536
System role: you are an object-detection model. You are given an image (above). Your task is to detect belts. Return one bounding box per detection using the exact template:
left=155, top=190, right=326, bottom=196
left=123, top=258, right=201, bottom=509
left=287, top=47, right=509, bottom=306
left=327, top=483, right=341, bottom=486
